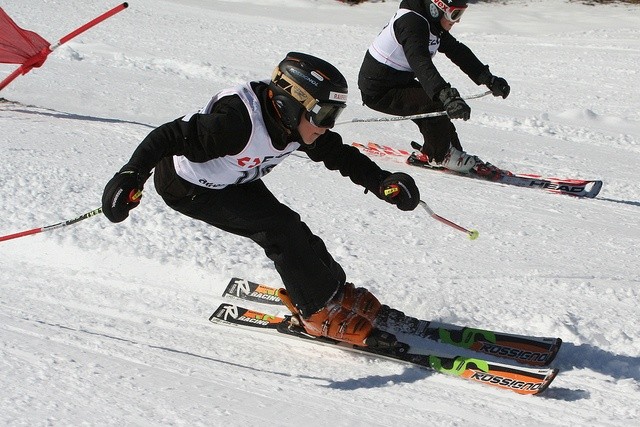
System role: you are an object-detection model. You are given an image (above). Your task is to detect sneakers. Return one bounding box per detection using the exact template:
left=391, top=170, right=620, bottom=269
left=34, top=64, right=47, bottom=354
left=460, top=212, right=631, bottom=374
left=333, top=283, right=381, bottom=323
left=427, top=141, right=482, bottom=171
left=303, top=301, right=372, bottom=347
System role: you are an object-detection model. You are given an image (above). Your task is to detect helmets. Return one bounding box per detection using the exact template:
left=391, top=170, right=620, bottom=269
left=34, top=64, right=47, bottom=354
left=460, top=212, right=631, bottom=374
left=268, top=52, right=348, bottom=128
left=426, top=0, right=468, bottom=22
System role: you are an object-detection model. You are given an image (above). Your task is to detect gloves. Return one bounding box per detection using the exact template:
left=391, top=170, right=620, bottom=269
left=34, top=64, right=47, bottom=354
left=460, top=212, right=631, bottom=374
left=378, top=173, right=420, bottom=211
left=102, top=165, right=150, bottom=223
left=439, top=88, right=471, bottom=121
left=477, top=67, right=508, bottom=96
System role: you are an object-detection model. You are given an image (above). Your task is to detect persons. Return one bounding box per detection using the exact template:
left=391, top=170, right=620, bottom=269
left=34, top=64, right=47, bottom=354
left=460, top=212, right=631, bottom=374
left=102, top=52, right=419, bottom=348
left=357, top=0, right=510, bottom=173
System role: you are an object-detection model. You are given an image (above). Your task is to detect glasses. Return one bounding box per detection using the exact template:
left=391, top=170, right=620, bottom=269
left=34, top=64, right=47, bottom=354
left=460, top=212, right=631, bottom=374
left=271, top=66, right=346, bottom=129
left=432, top=0, right=467, bottom=25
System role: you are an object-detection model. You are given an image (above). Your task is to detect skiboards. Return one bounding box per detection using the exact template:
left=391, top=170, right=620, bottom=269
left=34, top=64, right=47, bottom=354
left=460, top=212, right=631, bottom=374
left=351, top=141, right=602, bottom=198
left=210, top=278, right=562, bottom=396
